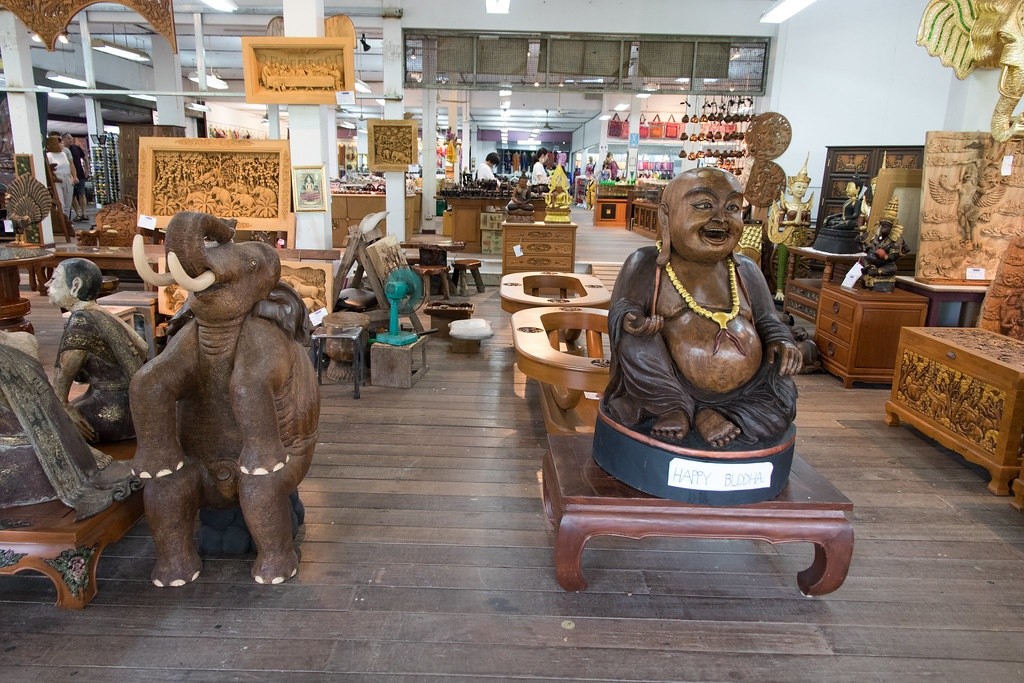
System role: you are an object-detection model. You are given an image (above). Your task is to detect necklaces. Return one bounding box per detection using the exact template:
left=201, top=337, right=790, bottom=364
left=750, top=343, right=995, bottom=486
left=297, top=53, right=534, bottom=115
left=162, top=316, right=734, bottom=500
left=656, top=241, right=746, bottom=355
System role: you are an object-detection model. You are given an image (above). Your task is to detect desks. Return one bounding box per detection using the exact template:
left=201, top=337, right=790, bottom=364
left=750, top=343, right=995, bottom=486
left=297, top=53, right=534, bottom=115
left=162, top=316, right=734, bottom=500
left=399, top=240, right=466, bottom=295
left=501, top=219, right=578, bottom=277
left=499, top=271, right=611, bottom=356
left=96, top=291, right=159, bottom=362
left=0, top=436, right=146, bottom=608
left=0, top=248, right=55, bottom=335
left=510, top=306, right=611, bottom=434
left=895, top=275, right=989, bottom=329
left=58, top=306, right=136, bottom=331
left=542, top=435, right=854, bottom=597
left=34, top=245, right=165, bottom=296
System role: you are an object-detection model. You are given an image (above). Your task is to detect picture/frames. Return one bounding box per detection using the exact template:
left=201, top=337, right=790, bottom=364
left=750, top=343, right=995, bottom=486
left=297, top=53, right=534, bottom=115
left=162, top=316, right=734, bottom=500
left=292, top=165, right=327, bottom=211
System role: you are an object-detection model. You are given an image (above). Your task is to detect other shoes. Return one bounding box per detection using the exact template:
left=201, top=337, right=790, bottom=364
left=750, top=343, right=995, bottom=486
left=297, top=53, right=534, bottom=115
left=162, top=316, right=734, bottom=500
left=80, top=213, right=89, bottom=220
left=74, top=217, right=82, bottom=222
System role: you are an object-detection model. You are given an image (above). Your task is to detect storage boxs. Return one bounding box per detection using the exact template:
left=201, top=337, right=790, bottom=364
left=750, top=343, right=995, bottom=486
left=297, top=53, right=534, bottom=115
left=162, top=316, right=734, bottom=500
left=480, top=213, right=502, bottom=230
left=481, top=230, right=502, bottom=254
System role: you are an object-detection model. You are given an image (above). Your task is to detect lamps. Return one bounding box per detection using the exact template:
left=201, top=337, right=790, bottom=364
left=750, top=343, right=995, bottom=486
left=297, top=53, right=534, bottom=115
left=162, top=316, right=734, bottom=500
left=188, top=35, right=228, bottom=90
left=359, top=33, right=370, bottom=51
left=200, top=0, right=239, bottom=13
left=354, top=70, right=371, bottom=94
left=91, top=8, right=150, bottom=64
left=339, top=98, right=368, bottom=140
left=45, top=35, right=87, bottom=87
left=128, top=64, right=156, bottom=101
left=184, top=98, right=211, bottom=112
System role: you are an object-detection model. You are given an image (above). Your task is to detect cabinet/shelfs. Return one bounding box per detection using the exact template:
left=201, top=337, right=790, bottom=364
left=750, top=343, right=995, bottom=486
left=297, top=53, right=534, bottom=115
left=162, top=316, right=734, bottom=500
left=813, top=281, right=929, bottom=389
left=813, top=145, right=924, bottom=246
left=886, top=326, right=1024, bottom=496
left=783, top=247, right=867, bottom=324
left=451, top=204, right=481, bottom=252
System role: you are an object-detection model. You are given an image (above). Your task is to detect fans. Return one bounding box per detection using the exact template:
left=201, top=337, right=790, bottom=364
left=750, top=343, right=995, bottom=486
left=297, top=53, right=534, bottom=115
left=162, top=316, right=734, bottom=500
left=376, top=264, right=426, bottom=346
left=533, top=109, right=560, bottom=130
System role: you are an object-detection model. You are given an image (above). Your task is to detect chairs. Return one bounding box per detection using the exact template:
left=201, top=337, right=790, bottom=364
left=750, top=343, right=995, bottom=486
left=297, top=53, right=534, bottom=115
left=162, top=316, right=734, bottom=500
left=75, top=203, right=154, bottom=277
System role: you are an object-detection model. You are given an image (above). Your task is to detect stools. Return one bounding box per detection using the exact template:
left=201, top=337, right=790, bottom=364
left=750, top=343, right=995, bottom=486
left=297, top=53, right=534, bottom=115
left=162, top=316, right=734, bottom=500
left=311, top=327, right=365, bottom=399
left=412, top=265, right=461, bottom=303
left=451, top=259, right=485, bottom=297
left=370, top=335, right=429, bottom=388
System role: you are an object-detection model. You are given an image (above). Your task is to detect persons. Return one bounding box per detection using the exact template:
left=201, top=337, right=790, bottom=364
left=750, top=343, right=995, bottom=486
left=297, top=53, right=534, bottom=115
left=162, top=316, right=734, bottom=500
left=478, top=152, right=501, bottom=186
left=856, top=195, right=904, bottom=276
left=508, top=174, right=535, bottom=210
left=549, top=177, right=569, bottom=208
left=605, top=168, right=803, bottom=447
left=532, top=148, right=552, bottom=184
left=775, top=152, right=811, bottom=300
left=46, top=131, right=90, bottom=227
left=940, top=166, right=1000, bottom=241
left=822, top=166, right=862, bottom=230
left=585, top=156, right=596, bottom=175
left=600, top=164, right=612, bottom=181
left=44, top=258, right=148, bottom=445
left=586, top=175, right=596, bottom=210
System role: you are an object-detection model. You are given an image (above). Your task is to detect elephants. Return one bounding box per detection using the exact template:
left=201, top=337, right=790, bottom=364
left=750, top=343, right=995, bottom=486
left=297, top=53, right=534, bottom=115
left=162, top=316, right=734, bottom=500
left=127, top=211, right=323, bottom=587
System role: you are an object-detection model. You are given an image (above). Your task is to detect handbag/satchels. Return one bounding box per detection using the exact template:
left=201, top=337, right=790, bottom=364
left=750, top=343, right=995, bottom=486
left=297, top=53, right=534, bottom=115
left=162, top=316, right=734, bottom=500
left=609, top=112, right=678, bottom=139
